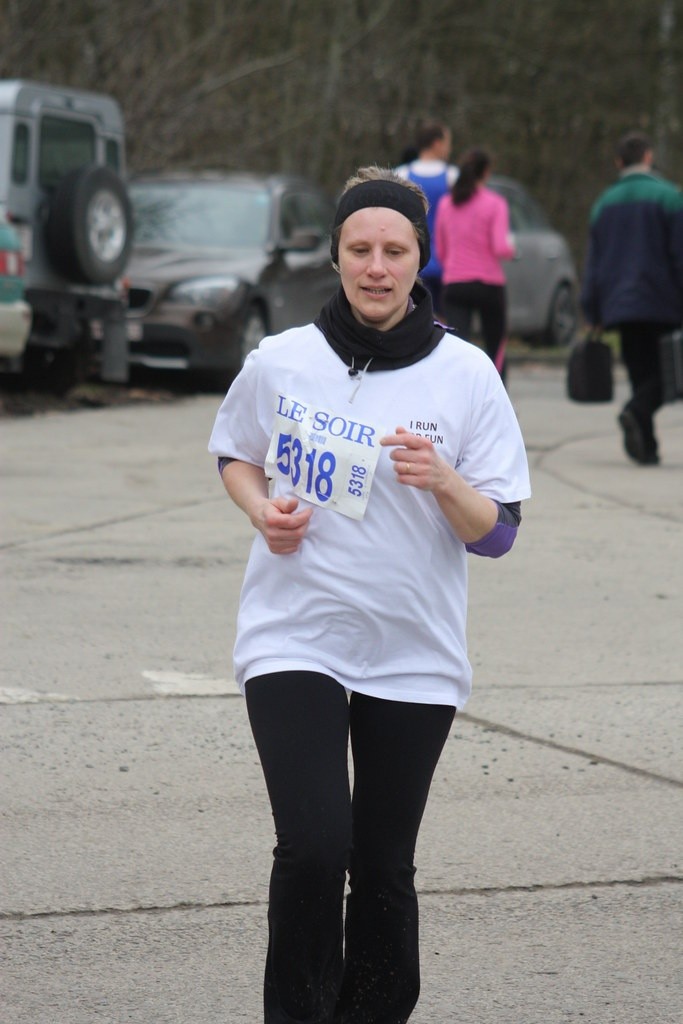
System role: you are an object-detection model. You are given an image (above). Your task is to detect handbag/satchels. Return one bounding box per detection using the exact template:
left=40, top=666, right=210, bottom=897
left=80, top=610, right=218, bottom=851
left=565, top=325, right=614, bottom=403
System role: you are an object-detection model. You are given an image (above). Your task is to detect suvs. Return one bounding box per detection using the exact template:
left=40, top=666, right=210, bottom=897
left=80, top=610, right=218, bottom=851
left=0, top=83, right=137, bottom=392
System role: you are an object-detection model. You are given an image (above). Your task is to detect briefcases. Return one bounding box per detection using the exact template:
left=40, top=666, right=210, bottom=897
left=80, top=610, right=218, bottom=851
left=660, top=327, right=683, bottom=403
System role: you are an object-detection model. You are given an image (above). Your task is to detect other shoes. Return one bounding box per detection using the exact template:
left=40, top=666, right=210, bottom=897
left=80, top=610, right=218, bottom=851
left=617, top=398, right=660, bottom=464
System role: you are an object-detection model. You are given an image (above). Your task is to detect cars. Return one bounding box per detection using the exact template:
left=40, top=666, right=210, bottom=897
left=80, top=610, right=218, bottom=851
left=485, top=174, right=585, bottom=352
left=122, top=170, right=341, bottom=394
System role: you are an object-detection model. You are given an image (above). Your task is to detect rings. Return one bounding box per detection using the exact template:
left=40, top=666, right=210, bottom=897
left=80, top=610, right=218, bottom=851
left=406, top=463, right=410, bottom=474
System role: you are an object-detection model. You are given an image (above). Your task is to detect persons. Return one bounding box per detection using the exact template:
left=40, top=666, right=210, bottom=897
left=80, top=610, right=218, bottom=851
left=434, top=148, right=515, bottom=387
left=391, top=120, right=459, bottom=324
left=578, top=132, right=683, bottom=467
left=208, top=165, right=531, bottom=1023
